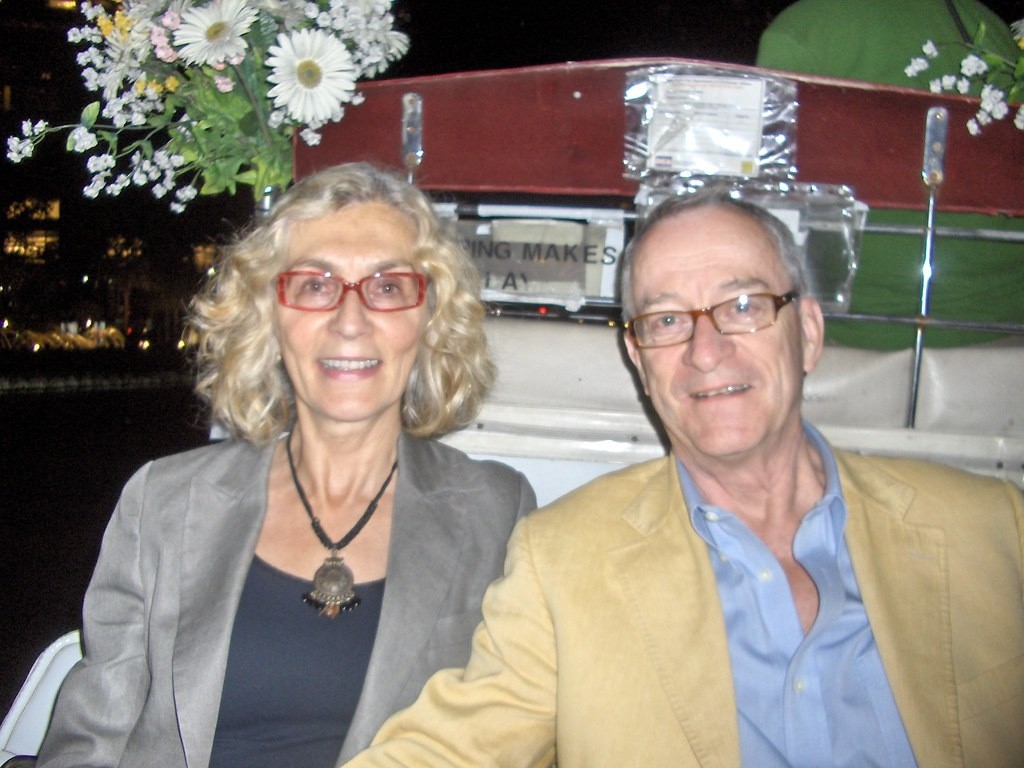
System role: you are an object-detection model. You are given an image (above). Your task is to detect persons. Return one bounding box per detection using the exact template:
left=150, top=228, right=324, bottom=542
left=38, top=160, right=539, bottom=768
left=339, top=189, right=1024, bottom=768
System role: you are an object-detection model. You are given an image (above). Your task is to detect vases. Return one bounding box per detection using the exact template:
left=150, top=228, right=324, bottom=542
left=253, top=184, right=280, bottom=226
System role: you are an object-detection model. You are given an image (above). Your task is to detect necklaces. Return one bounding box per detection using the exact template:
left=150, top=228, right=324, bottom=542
left=286, top=432, right=398, bottom=620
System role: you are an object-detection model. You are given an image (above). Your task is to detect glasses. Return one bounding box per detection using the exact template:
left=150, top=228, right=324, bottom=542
left=278, top=271, right=427, bottom=313
left=630, top=289, right=799, bottom=349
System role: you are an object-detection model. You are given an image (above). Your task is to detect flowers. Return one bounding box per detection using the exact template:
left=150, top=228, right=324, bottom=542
left=903, top=18, right=1024, bottom=137
left=0, top=0, right=412, bottom=215
